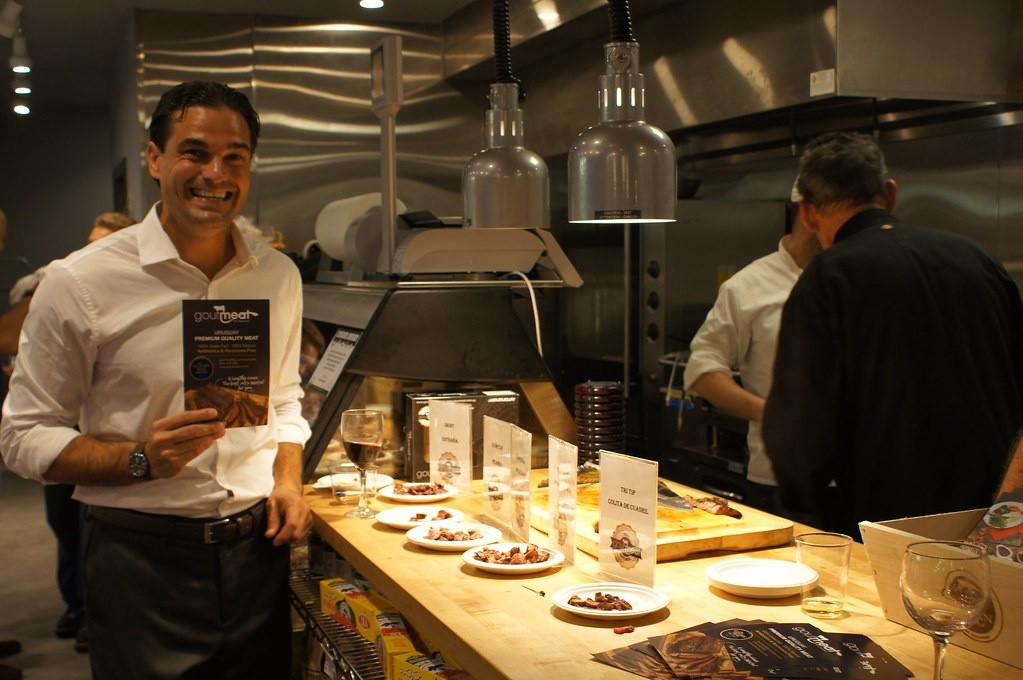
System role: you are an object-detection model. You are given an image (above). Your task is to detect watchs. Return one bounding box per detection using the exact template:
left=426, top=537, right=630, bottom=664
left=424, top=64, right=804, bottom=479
left=128, top=441, right=151, bottom=483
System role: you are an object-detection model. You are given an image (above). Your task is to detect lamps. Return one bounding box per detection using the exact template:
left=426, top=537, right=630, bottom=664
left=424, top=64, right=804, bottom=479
left=0, top=1, right=23, bottom=39
left=569, top=0, right=678, bottom=221
left=7, top=28, right=31, bottom=72
left=13, top=94, right=30, bottom=114
left=10, top=71, right=32, bottom=94
left=460, top=2, right=551, bottom=229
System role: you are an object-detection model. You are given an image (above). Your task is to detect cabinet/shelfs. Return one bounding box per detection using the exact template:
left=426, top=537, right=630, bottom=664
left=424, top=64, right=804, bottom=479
left=282, top=411, right=1023, bottom=679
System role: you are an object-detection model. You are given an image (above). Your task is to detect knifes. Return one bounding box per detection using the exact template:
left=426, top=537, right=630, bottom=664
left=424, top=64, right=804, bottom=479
left=658, top=485, right=693, bottom=510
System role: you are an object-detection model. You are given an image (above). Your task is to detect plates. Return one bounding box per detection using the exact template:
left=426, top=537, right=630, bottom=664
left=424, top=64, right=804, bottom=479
left=378, top=483, right=459, bottom=502
left=461, top=543, right=564, bottom=575
left=553, top=582, right=671, bottom=621
left=376, top=506, right=465, bottom=530
left=407, top=522, right=503, bottom=552
left=316, top=472, right=394, bottom=497
left=982, top=501, right=1023, bottom=529
left=706, top=558, right=821, bottom=598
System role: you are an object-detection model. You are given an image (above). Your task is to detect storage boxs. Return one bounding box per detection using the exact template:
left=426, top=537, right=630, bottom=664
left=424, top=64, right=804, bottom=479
left=319, top=577, right=476, bottom=679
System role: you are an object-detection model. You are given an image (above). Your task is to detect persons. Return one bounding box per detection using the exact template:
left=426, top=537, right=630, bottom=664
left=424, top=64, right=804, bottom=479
left=0, top=80, right=313, bottom=680
left=0, top=208, right=139, bottom=652
left=757, top=132, right=1023, bottom=544
left=684, top=176, right=825, bottom=519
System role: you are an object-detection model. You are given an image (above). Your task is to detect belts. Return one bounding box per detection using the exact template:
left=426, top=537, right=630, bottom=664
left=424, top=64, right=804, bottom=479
left=87, top=500, right=265, bottom=544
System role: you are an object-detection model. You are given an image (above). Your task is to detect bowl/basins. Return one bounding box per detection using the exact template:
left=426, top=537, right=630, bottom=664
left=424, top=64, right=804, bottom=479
left=996, top=545, right=1012, bottom=558
left=1017, top=550, right=1023, bottom=563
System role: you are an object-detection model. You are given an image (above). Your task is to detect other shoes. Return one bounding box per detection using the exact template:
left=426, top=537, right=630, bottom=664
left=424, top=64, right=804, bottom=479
left=0, top=664, right=22, bottom=680
left=54, top=613, right=80, bottom=640
left=74, top=637, right=91, bottom=654
left=0, top=640, right=20, bottom=657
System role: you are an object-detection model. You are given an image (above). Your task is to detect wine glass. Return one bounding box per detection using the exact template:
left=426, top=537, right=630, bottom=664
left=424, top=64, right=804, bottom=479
left=899, top=540, right=991, bottom=680
left=339, top=410, right=383, bottom=517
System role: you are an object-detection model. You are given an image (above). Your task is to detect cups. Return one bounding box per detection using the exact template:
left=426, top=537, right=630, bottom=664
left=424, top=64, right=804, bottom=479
left=795, top=533, right=853, bottom=620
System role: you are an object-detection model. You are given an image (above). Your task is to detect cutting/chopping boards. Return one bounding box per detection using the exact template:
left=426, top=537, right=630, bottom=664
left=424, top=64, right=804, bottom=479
left=531, top=468, right=794, bottom=563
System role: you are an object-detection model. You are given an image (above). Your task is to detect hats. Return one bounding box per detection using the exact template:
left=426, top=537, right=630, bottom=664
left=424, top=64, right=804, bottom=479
left=790, top=173, right=804, bottom=202
